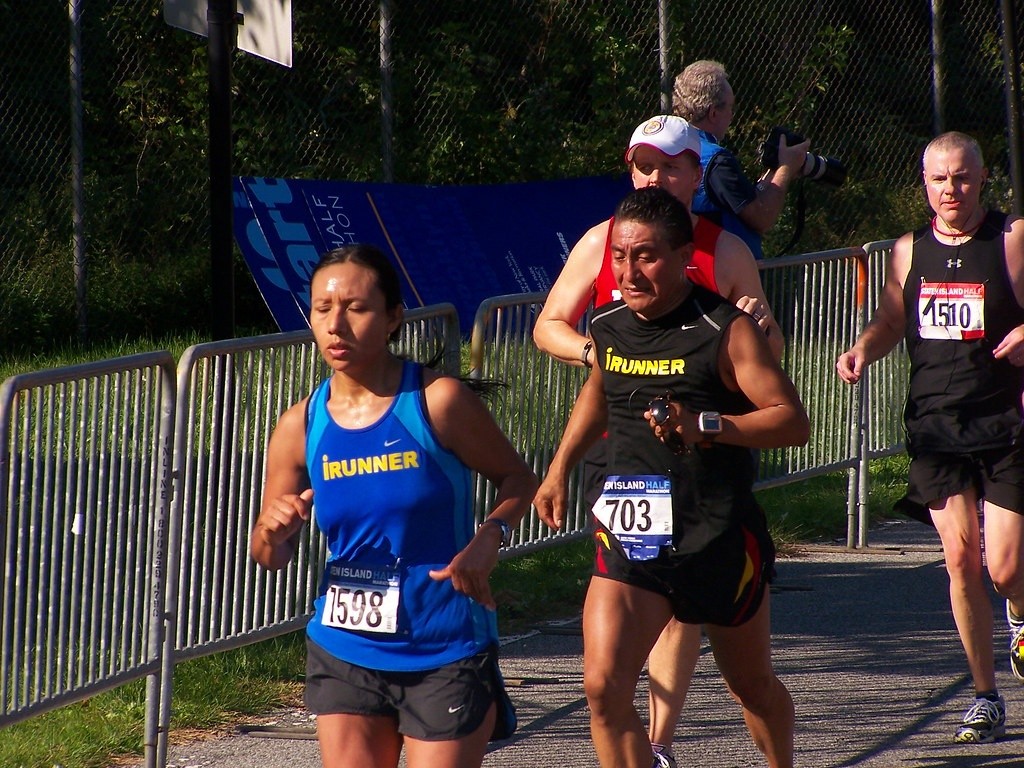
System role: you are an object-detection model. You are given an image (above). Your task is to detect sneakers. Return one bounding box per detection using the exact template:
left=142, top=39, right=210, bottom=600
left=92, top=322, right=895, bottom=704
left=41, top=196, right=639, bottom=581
left=954, top=695, right=1006, bottom=743
left=1006, top=597, right=1024, bottom=680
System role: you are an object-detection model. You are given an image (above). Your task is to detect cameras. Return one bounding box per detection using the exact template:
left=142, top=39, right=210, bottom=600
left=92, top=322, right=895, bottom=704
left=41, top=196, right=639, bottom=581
left=757, top=126, right=848, bottom=188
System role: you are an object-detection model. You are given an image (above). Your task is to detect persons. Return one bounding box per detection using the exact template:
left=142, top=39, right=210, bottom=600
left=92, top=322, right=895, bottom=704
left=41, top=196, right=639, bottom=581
left=672, top=59, right=810, bottom=293
left=252, top=244, right=540, bottom=767
left=533, top=114, right=790, bottom=766
left=837, top=130, right=1023, bottom=742
left=533, top=186, right=811, bottom=767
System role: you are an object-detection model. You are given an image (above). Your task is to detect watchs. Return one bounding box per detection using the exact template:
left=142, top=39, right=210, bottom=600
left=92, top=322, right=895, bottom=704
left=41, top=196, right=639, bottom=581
left=477, top=518, right=511, bottom=549
left=699, top=409, right=723, bottom=448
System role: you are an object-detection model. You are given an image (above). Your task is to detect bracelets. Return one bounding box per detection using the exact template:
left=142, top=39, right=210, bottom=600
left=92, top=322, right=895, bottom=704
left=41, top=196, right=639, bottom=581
left=581, top=338, right=595, bottom=370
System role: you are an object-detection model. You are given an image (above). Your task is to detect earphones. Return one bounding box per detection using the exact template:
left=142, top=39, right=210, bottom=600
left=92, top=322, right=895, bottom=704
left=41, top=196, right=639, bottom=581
left=924, top=183, right=927, bottom=187
left=981, top=176, right=985, bottom=188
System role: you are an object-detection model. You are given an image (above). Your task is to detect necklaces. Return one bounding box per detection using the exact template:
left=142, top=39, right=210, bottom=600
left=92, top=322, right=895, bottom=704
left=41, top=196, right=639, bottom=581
left=931, top=208, right=986, bottom=243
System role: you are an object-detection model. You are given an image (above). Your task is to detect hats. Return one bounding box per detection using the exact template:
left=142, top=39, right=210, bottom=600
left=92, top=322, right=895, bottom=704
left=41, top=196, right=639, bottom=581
left=625, top=115, right=701, bottom=162
